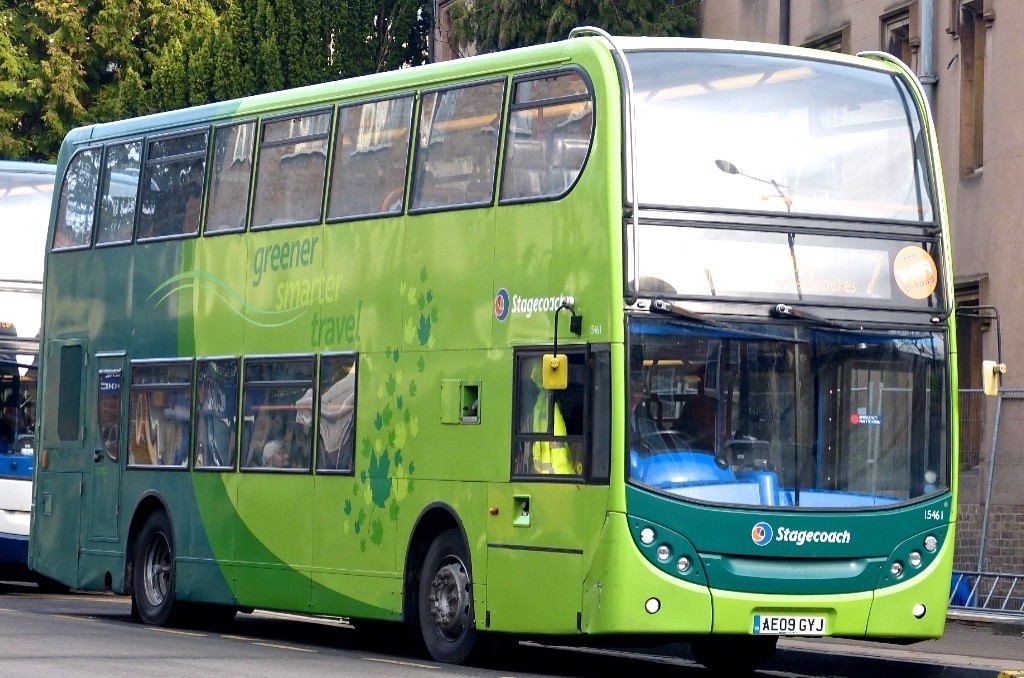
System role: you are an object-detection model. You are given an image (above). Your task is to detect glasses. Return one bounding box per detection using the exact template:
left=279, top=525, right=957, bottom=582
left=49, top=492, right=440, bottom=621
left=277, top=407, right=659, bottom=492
left=629, top=386, right=647, bottom=396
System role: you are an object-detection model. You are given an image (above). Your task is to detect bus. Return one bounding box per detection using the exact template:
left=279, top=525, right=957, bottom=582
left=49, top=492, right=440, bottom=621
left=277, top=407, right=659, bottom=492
left=0, top=159, right=160, bottom=597
left=30, top=26, right=1006, bottom=678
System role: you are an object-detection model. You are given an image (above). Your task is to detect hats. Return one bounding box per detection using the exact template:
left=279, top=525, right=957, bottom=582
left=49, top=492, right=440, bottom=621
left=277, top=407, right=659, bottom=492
left=2, top=393, right=31, bottom=407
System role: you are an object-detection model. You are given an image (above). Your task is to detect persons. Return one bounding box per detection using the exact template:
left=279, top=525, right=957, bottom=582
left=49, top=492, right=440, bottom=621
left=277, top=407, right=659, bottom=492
left=629, top=376, right=663, bottom=458
left=261, top=439, right=291, bottom=469
left=0, top=392, right=33, bottom=451
